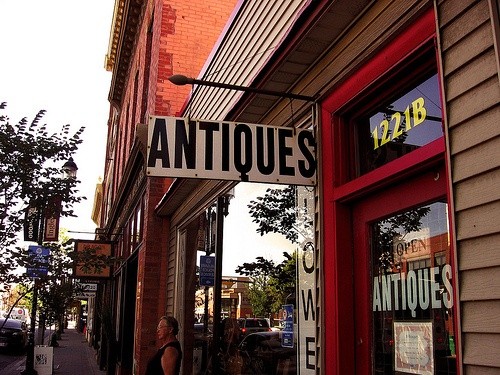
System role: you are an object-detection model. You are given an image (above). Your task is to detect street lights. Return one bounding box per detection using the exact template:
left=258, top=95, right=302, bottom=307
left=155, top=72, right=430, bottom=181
left=21, top=156, right=78, bottom=375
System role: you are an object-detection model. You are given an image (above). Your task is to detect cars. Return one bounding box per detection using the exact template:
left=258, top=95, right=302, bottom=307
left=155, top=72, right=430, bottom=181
left=0, top=317, right=29, bottom=356
left=220, top=316, right=297, bottom=375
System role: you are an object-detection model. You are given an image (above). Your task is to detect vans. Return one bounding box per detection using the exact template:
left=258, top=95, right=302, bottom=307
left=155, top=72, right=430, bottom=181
left=5, top=305, right=29, bottom=329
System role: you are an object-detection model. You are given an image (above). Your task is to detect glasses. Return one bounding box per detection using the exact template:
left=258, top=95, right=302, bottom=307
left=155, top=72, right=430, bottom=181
left=157, top=325, right=170, bottom=330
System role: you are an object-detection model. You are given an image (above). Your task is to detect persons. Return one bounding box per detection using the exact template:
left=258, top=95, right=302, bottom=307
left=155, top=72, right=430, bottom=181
left=146, top=316, right=182, bottom=375
